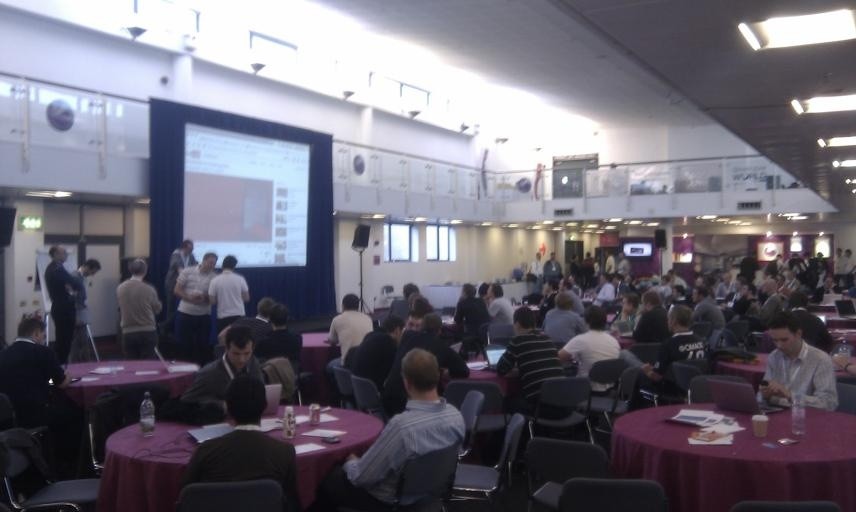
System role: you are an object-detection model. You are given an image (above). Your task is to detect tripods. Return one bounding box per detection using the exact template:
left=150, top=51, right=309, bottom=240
left=358, top=250, right=372, bottom=315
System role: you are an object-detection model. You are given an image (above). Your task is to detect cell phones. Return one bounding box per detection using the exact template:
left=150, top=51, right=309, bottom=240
left=321, top=436, right=341, bottom=444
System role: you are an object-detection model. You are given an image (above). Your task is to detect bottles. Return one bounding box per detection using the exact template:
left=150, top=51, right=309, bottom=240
left=309, top=403, right=320, bottom=426
left=140, top=392, right=154, bottom=437
left=839, top=338, right=851, bottom=362
left=283, top=406, right=296, bottom=439
left=792, top=394, right=805, bottom=435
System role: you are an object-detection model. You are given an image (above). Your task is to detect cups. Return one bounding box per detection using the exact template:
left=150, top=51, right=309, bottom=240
left=752, top=415, right=768, bottom=437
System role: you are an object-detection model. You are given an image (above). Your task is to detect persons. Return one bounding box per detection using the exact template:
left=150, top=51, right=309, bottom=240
left=160, top=238, right=855, bottom=511
left=0, top=317, right=99, bottom=476
left=71, top=257, right=101, bottom=361
left=116, top=257, right=163, bottom=359
left=45, top=245, right=88, bottom=362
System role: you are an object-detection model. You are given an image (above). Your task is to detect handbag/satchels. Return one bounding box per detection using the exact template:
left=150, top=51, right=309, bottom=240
left=458, top=337, right=487, bottom=360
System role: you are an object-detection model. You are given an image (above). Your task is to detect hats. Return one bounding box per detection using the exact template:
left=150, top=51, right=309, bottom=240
left=581, top=306, right=607, bottom=321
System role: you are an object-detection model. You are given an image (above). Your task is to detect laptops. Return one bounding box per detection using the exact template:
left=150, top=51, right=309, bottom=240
left=705, top=376, right=785, bottom=414
left=261, top=383, right=282, bottom=416
left=154, top=345, right=200, bottom=373
left=483, top=345, right=508, bottom=372
left=823, top=293, right=843, bottom=304
left=835, top=299, right=856, bottom=320
left=614, top=320, right=634, bottom=339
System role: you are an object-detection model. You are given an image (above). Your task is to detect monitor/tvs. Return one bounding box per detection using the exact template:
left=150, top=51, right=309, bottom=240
left=623, top=241, right=654, bottom=262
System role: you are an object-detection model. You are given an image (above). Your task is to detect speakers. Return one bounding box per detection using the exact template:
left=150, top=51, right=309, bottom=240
left=655, top=229, right=666, bottom=247
left=352, top=224, right=370, bottom=248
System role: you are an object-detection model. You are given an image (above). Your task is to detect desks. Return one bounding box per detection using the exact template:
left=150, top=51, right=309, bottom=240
left=718, top=353, right=855, bottom=394
left=301, top=332, right=341, bottom=406
left=513, top=305, right=540, bottom=325
left=442, top=351, right=522, bottom=414
left=764, top=327, right=856, bottom=355
left=97, top=404, right=385, bottom=511
left=814, top=311, right=855, bottom=325
left=611, top=329, right=633, bottom=348
left=613, top=403, right=856, bottom=512
left=57, top=362, right=201, bottom=417
left=442, top=315, right=463, bottom=343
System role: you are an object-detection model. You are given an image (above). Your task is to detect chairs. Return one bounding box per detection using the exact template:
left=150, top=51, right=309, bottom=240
left=630, top=342, right=662, bottom=364
left=835, top=299, right=855, bottom=317
left=730, top=499, right=840, bottom=512
left=457, top=390, right=485, bottom=462
left=580, top=366, right=639, bottom=436
left=489, top=323, right=513, bottom=345
left=836, top=376, right=856, bottom=415
left=392, top=437, right=458, bottom=512
left=174, top=479, right=286, bottom=512
left=692, top=321, right=713, bottom=338
left=523, top=378, right=593, bottom=444
left=715, top=319, right=753, bottom=349
left=447, top=381, right=512, bottom=429
left=0, top=393, right=17, bottom=430
left=525, top=438, right=608, bottom=511
left=687, top=375, right=747, bottom=404
left=0, top=428, right=101, bottom=512
left=590, top=359, right=628, bottom=385
left=332, top=366, right=355, bottom=409
left=447, top=411, right=524, bottom=509
left=89, top=395, right=127, bottom=478
left=557, top=478, right=665, bottom=511
left=350, top=375, right=386, bottom=425
